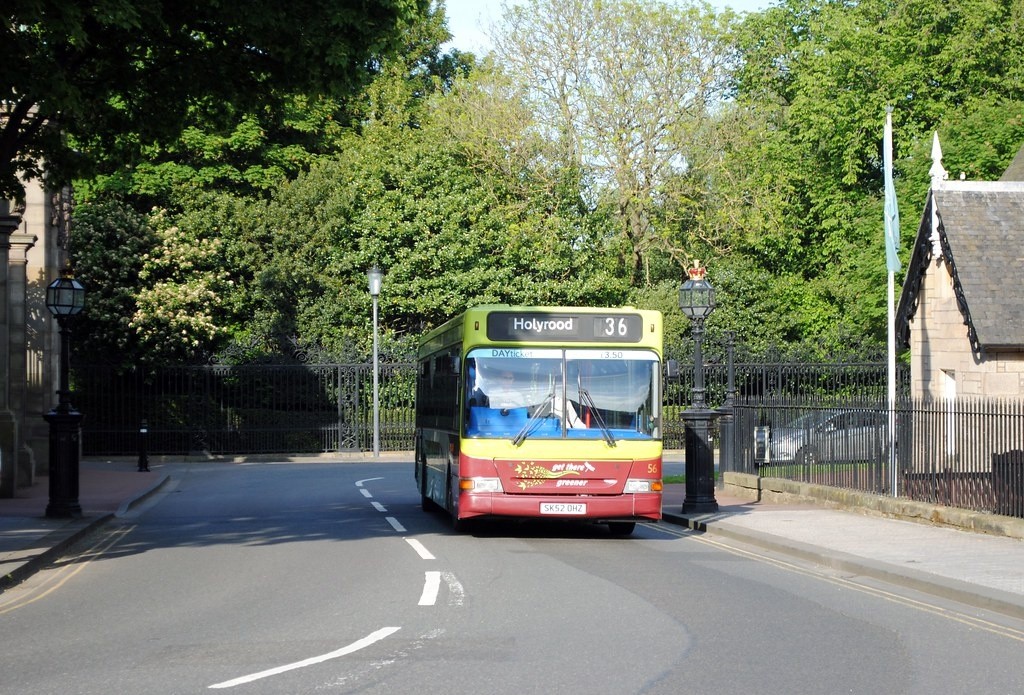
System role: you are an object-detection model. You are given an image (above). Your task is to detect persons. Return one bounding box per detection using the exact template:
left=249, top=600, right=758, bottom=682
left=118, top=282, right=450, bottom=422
left=487, top=371, right=527, bottom=409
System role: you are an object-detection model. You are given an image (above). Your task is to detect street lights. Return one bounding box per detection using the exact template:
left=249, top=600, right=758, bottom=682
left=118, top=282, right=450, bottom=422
left=41, top=266, right=90, bottom=521
left=367, top=263, right=387, bottom=461
left=676, top=258, right=727, bottom=513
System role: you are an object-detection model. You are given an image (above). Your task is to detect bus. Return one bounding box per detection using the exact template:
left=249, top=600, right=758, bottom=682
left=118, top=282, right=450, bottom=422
left=411, top=303, right=664, bottom=537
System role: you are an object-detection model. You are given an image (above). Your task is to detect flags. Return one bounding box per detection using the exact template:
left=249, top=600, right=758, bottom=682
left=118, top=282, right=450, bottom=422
left=881, top=125, right=903, bottom=272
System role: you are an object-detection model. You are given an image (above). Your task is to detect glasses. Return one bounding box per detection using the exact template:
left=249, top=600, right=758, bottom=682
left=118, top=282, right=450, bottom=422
left=500, top=376, right=514, bottom=380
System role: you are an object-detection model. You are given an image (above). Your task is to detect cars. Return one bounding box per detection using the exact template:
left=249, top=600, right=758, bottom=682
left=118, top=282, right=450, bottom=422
left=748, top=408, right=888, bottom=467
